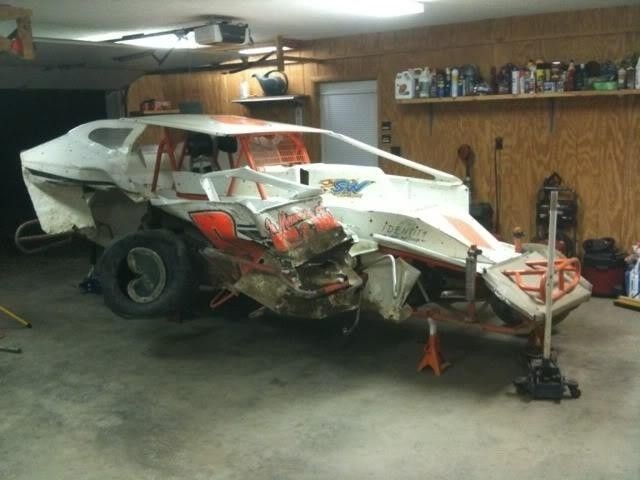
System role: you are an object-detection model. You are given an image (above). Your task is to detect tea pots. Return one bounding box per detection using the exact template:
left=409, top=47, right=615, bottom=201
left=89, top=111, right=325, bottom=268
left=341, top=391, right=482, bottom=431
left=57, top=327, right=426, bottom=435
left=251, top=70, right=289, bottom=94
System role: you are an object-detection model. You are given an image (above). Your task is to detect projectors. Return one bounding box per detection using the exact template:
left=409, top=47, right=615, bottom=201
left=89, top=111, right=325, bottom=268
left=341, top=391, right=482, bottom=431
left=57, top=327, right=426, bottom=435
left=194, top=24, right=249, bottom=46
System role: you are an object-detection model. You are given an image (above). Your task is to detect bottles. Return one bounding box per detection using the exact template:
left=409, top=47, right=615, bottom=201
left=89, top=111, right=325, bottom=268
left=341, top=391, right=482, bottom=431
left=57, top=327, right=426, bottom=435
left=140, top=98, right=172, bottom=112
left=416, top=60, right=638, bottom=98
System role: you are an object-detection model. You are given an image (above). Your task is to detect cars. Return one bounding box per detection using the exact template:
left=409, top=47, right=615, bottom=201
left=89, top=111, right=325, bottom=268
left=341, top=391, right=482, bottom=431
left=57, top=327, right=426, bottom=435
left=11, top=115, right=596, bottom=362
